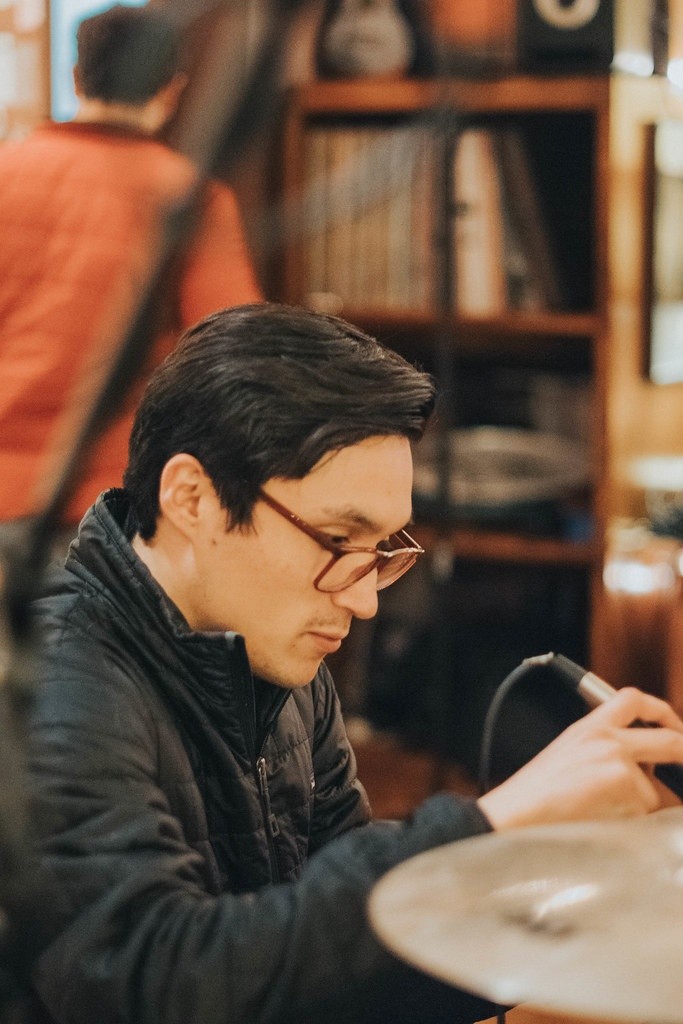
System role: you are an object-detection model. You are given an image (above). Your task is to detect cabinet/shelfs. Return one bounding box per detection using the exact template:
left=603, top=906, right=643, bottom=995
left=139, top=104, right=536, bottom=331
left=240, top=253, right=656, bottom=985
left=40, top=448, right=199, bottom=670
left=264, top=0, right=683, bottom=815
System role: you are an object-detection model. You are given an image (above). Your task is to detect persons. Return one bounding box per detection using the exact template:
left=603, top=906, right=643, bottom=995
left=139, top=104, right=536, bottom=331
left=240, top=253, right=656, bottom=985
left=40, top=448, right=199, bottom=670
left=0, top=302, right=683, bottom=1023
left=0, top=10, right=274, bottom=580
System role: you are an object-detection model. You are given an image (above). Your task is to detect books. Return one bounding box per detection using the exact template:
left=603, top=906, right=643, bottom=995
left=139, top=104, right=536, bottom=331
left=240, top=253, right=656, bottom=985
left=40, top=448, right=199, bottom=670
left=302, top=128, right=529, bottom=320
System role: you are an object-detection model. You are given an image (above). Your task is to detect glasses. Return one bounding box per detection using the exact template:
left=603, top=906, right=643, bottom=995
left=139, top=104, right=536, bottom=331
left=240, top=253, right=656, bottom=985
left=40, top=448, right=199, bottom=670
left=245, top=484, right=425, bottom=594
